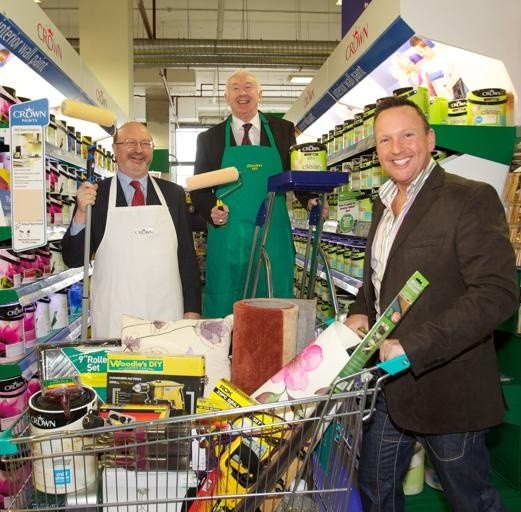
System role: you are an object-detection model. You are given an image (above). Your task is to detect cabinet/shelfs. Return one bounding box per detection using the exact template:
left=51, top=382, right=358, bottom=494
left=0, top=0, right=123, bottom=512
left=282, top=0, right=521, bottom=512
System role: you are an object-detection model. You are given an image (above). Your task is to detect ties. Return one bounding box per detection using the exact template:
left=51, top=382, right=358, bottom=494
left=241, top=123, right=252, bottom=144
left=129, top=181, right=145, bottom=206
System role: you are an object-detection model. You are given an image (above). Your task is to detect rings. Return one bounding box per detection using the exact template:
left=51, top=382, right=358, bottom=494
left=219, top=219, right=223, bottom=223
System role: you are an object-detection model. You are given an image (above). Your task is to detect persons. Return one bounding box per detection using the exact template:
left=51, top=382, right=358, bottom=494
left=343, top=98, right=521, bottom=512
left=189, top=70, right=329, bottom=320
left=61, top=122, right=200, bottom=340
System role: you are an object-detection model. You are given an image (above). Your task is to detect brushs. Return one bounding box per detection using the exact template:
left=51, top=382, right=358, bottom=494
left=186, top=166, right=242, bottom=211
left=61, top=99, right=117, bottom=342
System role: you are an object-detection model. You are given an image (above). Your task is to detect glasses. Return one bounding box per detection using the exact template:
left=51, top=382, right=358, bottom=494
left=114, top=138, right=154, bottom=149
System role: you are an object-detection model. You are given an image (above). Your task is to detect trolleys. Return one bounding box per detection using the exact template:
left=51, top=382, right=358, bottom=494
left=0, top=319, right=412, bottom=512
